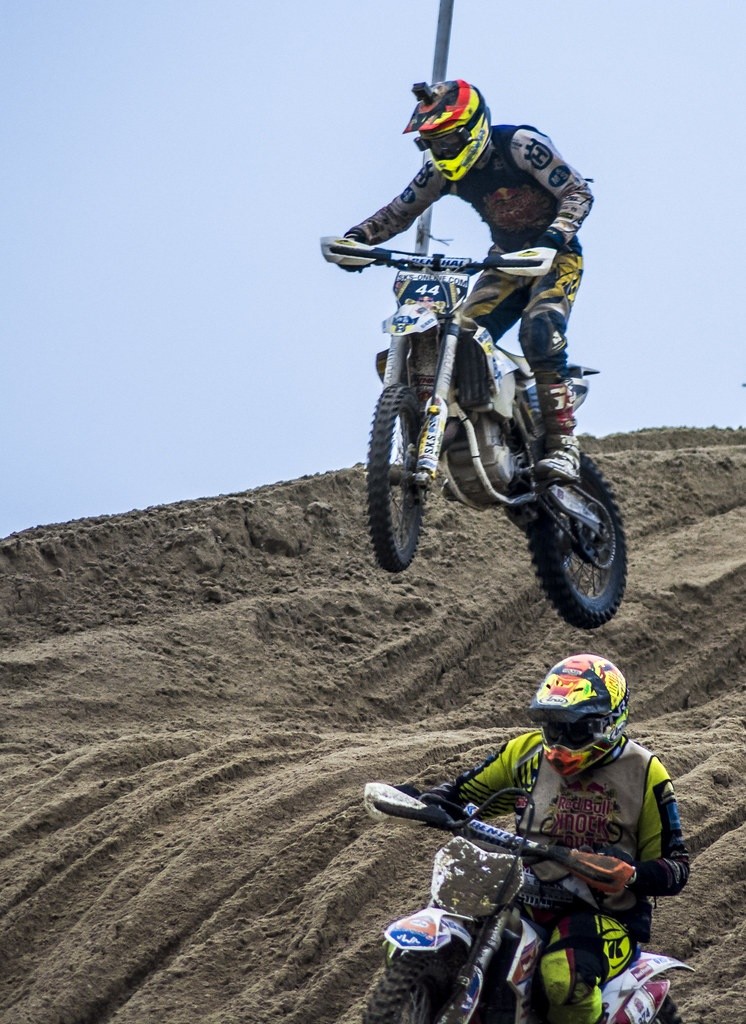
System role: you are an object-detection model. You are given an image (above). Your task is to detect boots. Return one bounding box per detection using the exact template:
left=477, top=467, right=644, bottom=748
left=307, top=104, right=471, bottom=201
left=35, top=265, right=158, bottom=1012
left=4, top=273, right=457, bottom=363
left=534, top=372, right=583, bottom=485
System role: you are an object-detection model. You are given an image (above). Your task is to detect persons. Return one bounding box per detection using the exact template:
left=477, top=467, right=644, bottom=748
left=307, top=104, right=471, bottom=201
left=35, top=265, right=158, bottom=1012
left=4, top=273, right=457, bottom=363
left=332, top=77, right=595, bottom=482
left=423, top=651, right=694, bottom=1024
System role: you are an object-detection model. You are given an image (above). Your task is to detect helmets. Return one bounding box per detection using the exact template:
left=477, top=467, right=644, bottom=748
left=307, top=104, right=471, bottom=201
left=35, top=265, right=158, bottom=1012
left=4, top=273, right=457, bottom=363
left=402, top=80, right=493, bottom=181
left=527, top=655, right=629, bottom=784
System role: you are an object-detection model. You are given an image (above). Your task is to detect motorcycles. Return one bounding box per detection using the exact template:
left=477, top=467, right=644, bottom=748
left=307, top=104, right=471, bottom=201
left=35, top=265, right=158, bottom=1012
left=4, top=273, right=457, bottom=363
left=319, top=236, right=630, bottom=630
left=360, top=781, right=695, bottom=1024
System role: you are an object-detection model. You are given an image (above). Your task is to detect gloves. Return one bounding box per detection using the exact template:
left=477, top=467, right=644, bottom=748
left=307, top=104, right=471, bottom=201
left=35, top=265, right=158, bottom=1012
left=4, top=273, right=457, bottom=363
left=580, top=845, right=668, bottom=897
left=532, top=227, right=567, bottom=254
left=393, top=784, right=422, bottom=799
left=338, top=226, right=371, bottom=272
